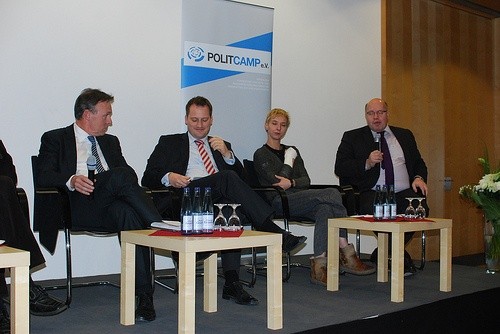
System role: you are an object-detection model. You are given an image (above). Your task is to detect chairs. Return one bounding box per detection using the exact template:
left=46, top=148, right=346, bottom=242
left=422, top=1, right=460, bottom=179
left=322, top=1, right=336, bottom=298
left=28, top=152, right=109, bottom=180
left=3, top=188, right=30, bottom=303
left=338, top=177, right=426, bottom=270
left=31, top=155, right=155, bottom=306
left=243, top=158, right=342, bottom=284
left=144, top=159, right=257, bottom=294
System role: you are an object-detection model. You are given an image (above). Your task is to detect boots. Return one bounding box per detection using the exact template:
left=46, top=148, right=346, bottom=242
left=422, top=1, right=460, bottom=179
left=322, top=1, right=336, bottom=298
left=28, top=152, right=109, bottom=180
left=310, top=257, right=327, bottom=286
left=339, top=244, right=376, bottom=275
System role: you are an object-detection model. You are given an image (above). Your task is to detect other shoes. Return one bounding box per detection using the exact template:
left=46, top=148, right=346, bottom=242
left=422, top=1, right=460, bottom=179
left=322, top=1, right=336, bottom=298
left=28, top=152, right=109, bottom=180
left=371, top=247, right=391, bottom=270
left=403, top=249, right=417, bottom=274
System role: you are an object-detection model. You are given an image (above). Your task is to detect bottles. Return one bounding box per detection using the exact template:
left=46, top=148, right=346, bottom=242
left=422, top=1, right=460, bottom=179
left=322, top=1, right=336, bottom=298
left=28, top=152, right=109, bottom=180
left=388, top=184, right=397, bottom=219
left=192, top=187, right=203, bottom=234
left=181, top=188, right=192, bottom=234
left=382, top=184, right=391, bottom=220
left=203, top=187, right=214, bottom=234
left=374, top=185, right=383, bottom=220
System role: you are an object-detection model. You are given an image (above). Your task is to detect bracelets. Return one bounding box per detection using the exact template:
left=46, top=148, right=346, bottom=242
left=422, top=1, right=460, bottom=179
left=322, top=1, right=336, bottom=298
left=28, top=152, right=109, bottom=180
left=288, top=178, right=295, bottom=187
left=415, top=177, right=424, bottom=181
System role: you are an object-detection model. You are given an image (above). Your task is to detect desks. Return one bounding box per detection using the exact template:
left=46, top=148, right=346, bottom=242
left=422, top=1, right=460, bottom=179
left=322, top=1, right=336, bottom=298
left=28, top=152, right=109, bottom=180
left=120, top=229, right=284, bottom=334
left=327, top=217, right=453, bottom=304
left=0, top=246, right=30, bottom=334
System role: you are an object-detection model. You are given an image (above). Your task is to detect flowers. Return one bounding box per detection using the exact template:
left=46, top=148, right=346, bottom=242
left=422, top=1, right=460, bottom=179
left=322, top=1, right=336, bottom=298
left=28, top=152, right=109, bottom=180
left=458, top=155, right=500, bottom=222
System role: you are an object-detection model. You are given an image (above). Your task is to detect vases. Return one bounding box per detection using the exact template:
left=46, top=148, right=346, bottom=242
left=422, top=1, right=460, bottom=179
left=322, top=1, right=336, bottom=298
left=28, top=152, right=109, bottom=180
left=483, top=213, right=500, bottom=276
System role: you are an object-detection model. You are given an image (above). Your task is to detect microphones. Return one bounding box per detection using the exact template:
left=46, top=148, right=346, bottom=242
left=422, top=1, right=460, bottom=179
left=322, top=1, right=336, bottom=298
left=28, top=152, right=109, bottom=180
left=374, top=133, right=381, bottom=167
left=87, top=156, right=97, bottom=200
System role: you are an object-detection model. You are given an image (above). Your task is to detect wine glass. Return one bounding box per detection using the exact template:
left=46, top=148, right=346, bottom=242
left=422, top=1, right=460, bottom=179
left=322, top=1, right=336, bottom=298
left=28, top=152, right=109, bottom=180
left=227, top=203, right=241, bottom=230
left=404, top=197, right=416, bottom=218
left=214, top=203, right=227, bottom=231
left=415, top=197, right=426, bottom=218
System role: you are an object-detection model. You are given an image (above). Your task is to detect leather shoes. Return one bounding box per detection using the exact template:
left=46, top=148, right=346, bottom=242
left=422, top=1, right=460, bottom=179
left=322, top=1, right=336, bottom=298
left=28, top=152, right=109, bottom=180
left=0, top=299, right=11, bottom=333
left=277, top=229, right=308, bottom=252
left=29, top=284, right=68, bottom=315
left=134, top=285, right=156, bottom=322
left=222, top=282, right=259, bottom=305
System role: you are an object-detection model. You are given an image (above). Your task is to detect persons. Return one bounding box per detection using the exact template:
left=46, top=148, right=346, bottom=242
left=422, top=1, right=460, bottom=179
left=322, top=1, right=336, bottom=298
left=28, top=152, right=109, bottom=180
left=34, top=88, right=168, bottom=322
left=141, top=96, right=307, bottom=306
left=334, top=97, right=430, bottom=274
left=0, top=137, right=70, bottom=334
left=253, top=108, right=377, bottom=287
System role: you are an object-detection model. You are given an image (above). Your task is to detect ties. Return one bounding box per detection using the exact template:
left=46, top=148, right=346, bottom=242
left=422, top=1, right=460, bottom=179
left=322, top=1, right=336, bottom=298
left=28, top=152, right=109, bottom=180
left=88, top=135, right=105, bottom=173
left=378, top=131, right=394, bottom=194
left=195, top=140, right=215, bottom=175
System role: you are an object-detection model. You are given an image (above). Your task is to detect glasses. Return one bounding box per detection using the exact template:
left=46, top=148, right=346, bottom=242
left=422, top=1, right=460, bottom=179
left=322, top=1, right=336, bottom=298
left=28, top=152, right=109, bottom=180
left=366, top=110, right=387, bottom=115
